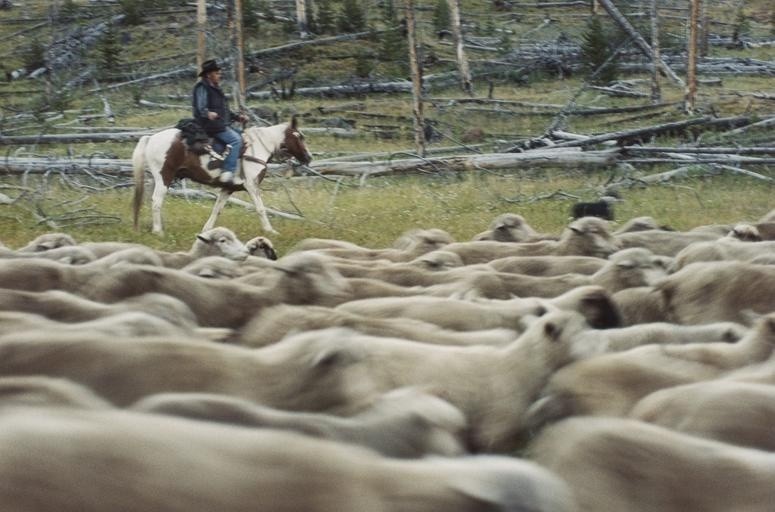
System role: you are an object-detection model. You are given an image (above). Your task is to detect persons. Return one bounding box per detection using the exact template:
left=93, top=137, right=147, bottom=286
left=191, top=58, right=250, bottom=186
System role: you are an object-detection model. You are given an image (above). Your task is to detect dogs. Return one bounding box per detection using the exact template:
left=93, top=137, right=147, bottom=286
left=570, top=195, right=616, bottom=223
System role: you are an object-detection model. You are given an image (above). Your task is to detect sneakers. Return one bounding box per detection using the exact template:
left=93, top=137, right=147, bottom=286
left=219, top=171, right=245, bottom=186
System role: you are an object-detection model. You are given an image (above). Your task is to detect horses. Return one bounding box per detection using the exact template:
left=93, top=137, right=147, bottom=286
left=129, top=114, right=314, bottom=238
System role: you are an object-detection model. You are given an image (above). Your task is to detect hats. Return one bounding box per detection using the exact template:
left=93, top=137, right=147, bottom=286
left=199, top=60, right=221, bottom=76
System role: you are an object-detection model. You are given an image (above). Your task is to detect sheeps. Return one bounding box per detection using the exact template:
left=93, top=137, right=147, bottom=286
left=0, top=205, right=775, bottom=512
